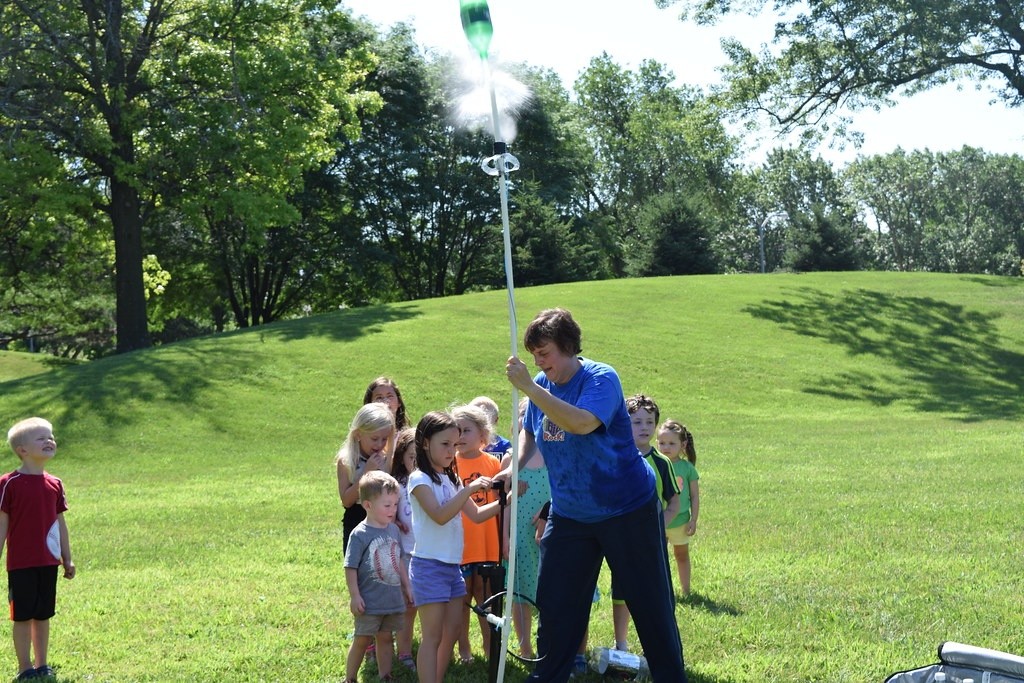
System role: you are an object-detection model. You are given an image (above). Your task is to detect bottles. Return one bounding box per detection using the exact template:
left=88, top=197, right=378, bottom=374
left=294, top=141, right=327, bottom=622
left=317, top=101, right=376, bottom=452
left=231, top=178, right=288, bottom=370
left=588, top=647, right=649, bottom=679
left=935, top=672, right=945, bottom=683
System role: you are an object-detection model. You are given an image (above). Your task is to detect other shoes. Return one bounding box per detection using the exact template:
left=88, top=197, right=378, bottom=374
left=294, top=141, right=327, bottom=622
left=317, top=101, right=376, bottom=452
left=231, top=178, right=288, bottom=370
left=36, top=665, right=55, bottom=677
left=573, top=654, right=587, bottom=674
left=20, top=668, right=39, bottom=679
left=380, top=672, right=398, bottom=683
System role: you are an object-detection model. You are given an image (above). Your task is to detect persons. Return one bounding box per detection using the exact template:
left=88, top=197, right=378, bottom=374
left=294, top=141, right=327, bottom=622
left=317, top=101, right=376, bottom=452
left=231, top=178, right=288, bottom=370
left=343, top=470, right=414, bottom=683
left=491, top=309, right=686, bottom=683
left=611, top=394, right=681, bottom=654
left=500, top=394, right=588, bottom=676
left=0, top=417, right=76, bottom=683
left=656, top=421, right=700, bottom=594
left=472, top=395, right=512, bottom=462
left=336, top=377, right=417, bottom=673
left=407, top=411, right=527, bottom=683
left=451, top=406, right=500, bottom=665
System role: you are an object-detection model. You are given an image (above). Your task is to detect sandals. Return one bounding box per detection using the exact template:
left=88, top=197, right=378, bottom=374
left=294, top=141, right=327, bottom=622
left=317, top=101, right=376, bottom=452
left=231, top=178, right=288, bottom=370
left=398, top=653, right=417, bottom=672
left=365, top=644, right=376, bottom=663
